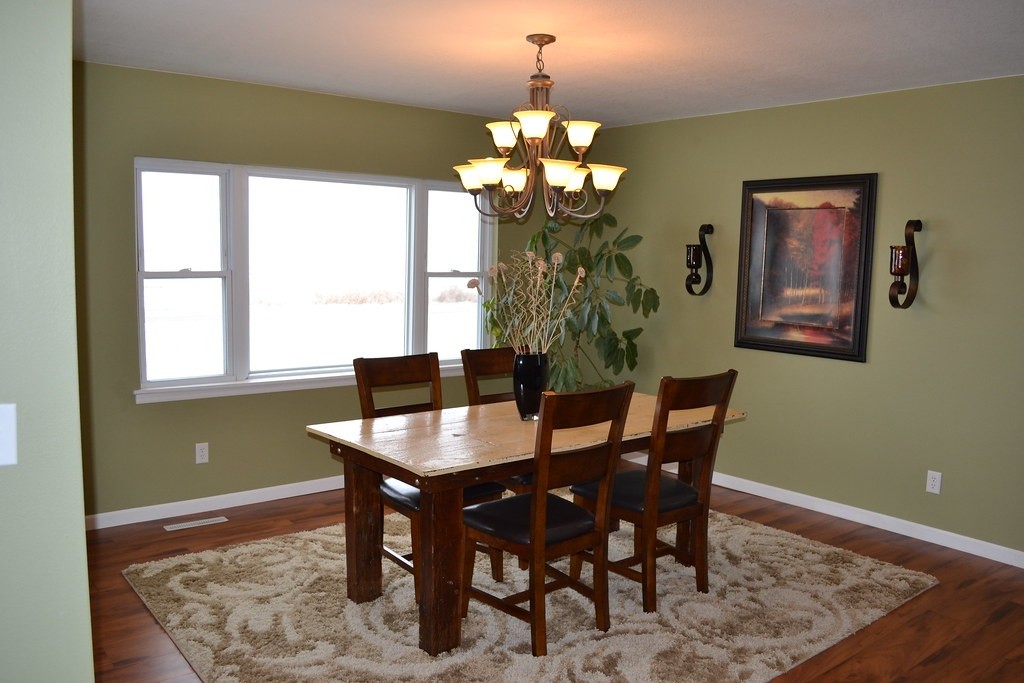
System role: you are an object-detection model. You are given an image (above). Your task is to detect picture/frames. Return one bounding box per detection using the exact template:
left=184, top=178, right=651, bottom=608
left=735, top=173, right=877, bottom=363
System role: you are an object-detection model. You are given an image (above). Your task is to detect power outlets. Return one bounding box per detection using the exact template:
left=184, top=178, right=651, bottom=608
left=927, top=469, right=942, bottom=495
left=195, top=440, right=209, bottom=462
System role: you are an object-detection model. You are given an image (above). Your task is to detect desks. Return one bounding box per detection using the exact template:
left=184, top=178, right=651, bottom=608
left=305, top=389, right=747, bottom=660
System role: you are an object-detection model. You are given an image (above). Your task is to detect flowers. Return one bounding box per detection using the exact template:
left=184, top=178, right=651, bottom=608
left=467, top=251, right=586, bottom=355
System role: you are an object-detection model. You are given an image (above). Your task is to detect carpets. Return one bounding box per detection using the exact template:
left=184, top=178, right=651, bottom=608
left=122, top=471, right=941, bottom=683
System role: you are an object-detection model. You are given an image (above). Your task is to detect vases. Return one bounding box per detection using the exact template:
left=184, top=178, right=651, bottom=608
left=514, top=351, right=549, bottom=419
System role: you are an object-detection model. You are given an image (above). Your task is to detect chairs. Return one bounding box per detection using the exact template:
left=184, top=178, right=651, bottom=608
left=461, top=344, right=559, bottom=570
left=353, top=353, right=503, bottom=618
left=567, top=367, right=739, bottom=615
left=456, top=378, right=635, bottom=658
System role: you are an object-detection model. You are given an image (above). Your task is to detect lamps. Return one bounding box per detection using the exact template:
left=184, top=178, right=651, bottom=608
left=684, top=223, right=716, bottom=295
left=888, top=219, right=923, bottom=310
left=453, top=33, right=627, bottom=220
